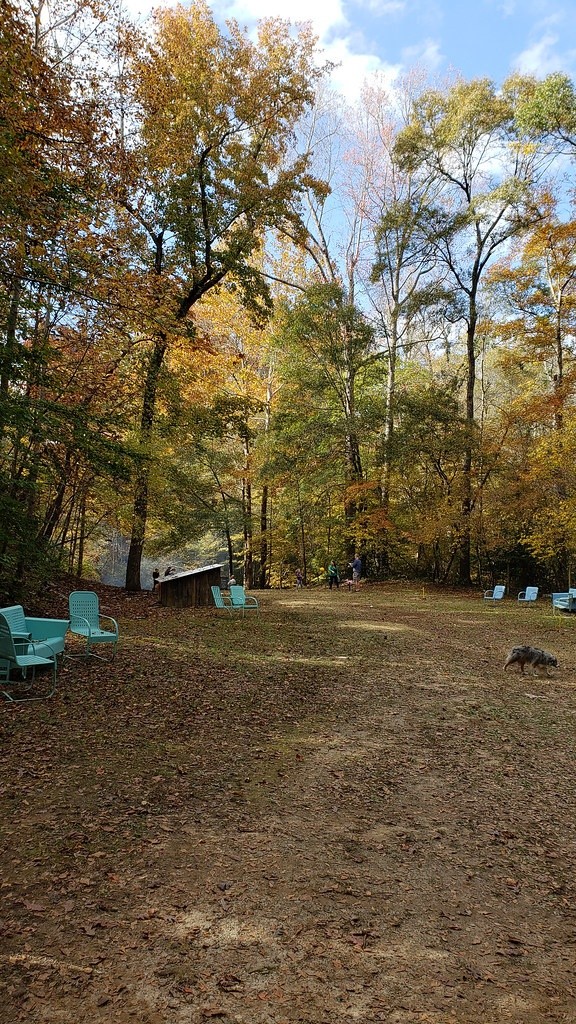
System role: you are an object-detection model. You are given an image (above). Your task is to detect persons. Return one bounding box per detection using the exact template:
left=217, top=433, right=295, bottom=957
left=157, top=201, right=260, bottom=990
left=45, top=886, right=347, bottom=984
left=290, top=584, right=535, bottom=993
left=296, top=553, right=362, bottom=592
left=228, top=575, right=236, bottom=586
left=153, top=567, right=176, bottom=588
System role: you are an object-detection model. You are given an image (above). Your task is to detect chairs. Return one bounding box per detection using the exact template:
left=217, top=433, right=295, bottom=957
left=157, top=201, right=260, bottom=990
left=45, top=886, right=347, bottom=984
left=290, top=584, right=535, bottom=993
left=211, top=586, right=240, bottom=618
left=0, top=611, right=57, bottom=704
left=230, top=586, right=259, bottom=617
left=552, top=588, right=576, bottom=615
left=484, top=586, right=505, bottom=608
left=518, top=587, right=538, bottom=608
left=0, top=605, right=71, bottom=684
left=63, top=591, right=118, bottom=665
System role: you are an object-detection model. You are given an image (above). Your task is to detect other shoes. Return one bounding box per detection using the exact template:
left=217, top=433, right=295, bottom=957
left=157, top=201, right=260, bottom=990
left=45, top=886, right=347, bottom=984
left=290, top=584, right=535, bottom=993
left=337, top=588, right=340, bottom=591
left=355, top=589, right=360, bottom=592
left=329, top=589, right=331, bottom=592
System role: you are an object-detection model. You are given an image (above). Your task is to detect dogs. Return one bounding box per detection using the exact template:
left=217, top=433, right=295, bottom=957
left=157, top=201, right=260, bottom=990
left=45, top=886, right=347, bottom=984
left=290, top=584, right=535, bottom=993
left=503, top=645, right=560, bottom=677
